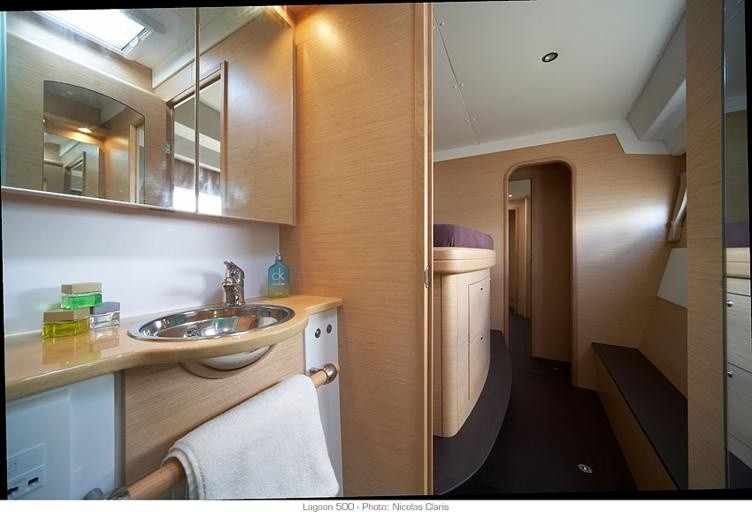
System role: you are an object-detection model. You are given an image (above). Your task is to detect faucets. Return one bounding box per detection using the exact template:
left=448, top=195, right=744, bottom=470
left=221, top=258, right=245, bottom=304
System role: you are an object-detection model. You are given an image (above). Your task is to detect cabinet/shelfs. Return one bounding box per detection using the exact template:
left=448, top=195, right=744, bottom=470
left=468, top=275, right=491, bottom=401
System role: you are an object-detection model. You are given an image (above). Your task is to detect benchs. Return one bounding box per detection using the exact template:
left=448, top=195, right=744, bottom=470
left=591, top=342, right=689, bottom=490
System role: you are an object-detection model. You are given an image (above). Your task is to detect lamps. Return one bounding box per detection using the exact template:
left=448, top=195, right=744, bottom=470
left=28, top=9, right=155, bottom=59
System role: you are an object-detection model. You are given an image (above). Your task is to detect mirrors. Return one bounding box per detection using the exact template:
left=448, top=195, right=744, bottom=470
left=0, top=5, right=298, bottom=228
left=718, top=0, right=752, bottom=489
left=42, top=79, right=146, bottom=203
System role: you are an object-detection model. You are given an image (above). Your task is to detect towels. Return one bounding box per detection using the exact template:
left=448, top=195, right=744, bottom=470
left=161, top=373, right=340, bottom=500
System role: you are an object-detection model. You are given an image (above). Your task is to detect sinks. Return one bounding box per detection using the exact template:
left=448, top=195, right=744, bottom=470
left=128, top=302, right=296, bottom=341
